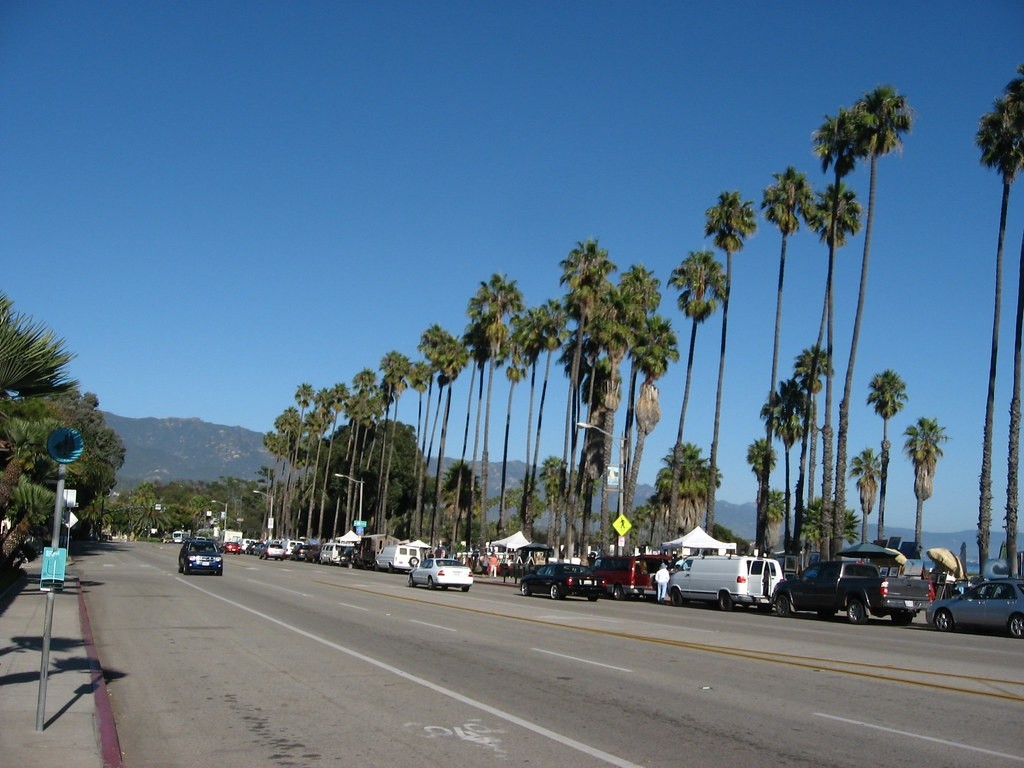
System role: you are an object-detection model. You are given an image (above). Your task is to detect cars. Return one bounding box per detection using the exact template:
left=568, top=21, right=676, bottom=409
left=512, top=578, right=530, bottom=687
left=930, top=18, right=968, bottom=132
left=925, top=579, right=1024, bottom=639
left=289, top=544, right=309, bottom=560
left=240, top=539, right=305, bottom=556
left=178, top=538, right=223, bottom=575
left=519, top=563, right=608, bottom=601
left=258, top=542, right=285, bottom=560
left=408, top=558, right=474, bottom=592
left=305, top=544, right=322, bottom=563
left=219, top=542, right=241, bottom=555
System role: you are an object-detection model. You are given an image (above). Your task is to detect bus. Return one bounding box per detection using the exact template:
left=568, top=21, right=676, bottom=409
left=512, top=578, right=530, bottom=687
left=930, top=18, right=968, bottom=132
left=173, top=531, right=190, bottom=543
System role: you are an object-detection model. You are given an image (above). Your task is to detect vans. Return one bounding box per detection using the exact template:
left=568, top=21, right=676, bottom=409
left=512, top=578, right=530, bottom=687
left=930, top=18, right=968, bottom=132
left=319, top=543, right=355, bottom=565
left=591, top=555, right=675, bottom=599
left=373, top=544, right=421, bottom=573
left=667, top=553, right=784, bottom=612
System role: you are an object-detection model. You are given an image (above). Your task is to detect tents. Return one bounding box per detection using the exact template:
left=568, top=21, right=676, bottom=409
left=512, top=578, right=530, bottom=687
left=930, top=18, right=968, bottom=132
left=335, top=531, right=361, bottom=544
left=491, top=531, right=530, bottom=562
left=661, top=525, right=737, bottom=556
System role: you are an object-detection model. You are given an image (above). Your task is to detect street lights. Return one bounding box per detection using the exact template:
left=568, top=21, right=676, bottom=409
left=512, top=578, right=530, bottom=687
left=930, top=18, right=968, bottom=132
left=334, top=473, right=364, bottom=535
left=253, top=491, right=274, bottom=538
left=576, top=422, right=628, bottom=556
left=211, top=500, right=228, bottom=528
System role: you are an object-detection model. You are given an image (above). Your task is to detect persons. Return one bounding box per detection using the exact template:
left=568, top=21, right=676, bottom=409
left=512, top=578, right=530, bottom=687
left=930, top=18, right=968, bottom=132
left=655, top=563, right=670, bottom=603
left=481, top=554, right=500, bottom=577
left=670, top=555, right=684, bottom=569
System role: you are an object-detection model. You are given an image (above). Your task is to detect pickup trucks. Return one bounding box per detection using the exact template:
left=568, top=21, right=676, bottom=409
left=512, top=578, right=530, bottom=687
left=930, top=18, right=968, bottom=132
left=769, top=560, right=936, bottom=625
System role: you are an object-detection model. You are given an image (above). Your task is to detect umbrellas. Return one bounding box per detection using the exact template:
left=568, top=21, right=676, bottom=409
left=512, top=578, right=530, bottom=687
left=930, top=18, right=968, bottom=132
left=518, top=542, right=553, bottom=566
left=870, top=547, right=907, bottom=566
left=406, top=539, right=431, bottom=550
left=835, top=542, right=900, bottom=563
left=926, top=548, right=963, bottom=578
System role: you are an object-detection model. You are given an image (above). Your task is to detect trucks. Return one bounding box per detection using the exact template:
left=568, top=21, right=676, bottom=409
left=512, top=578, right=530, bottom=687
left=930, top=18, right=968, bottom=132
left=222, top=530, right=244, bottom=544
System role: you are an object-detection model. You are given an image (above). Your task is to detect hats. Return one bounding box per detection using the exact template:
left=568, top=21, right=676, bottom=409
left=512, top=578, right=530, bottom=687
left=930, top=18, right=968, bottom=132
left=660, top=563, right=667, bottom=569
